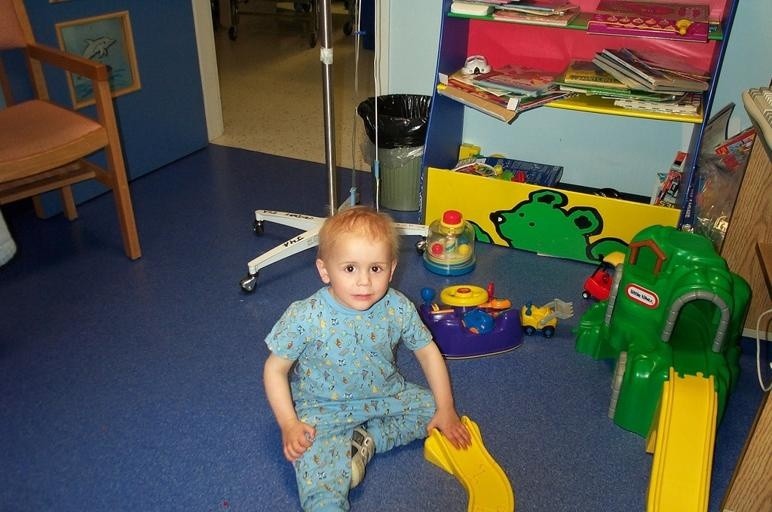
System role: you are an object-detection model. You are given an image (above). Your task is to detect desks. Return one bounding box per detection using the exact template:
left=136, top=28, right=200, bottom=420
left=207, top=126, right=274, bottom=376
left=707, top=86, right=772, bottom=512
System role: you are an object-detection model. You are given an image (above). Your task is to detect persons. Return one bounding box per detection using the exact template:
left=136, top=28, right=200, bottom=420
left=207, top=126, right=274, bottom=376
left=262, top=202, right=475, bottom=512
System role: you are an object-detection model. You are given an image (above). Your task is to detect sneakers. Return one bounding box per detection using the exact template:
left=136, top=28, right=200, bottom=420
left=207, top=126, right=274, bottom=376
left=349, top=422, right=375, bottom=491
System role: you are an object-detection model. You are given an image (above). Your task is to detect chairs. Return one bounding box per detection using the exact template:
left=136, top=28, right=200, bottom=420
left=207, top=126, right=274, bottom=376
left=0, top=0, right=143, bottom=261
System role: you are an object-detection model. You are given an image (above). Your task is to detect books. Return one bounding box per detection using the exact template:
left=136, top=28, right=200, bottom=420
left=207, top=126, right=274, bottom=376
left=436, top=0, right=722, bottom=124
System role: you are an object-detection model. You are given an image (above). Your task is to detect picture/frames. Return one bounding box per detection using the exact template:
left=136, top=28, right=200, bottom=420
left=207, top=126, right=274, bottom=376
left=55, top=10, right=143, bottom=111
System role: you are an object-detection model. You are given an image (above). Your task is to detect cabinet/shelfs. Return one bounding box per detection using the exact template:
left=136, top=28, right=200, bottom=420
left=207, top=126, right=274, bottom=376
left=418, top=0, right=738, bottom=269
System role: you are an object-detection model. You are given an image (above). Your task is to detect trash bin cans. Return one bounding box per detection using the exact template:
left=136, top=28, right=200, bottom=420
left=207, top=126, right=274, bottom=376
left=357, top=94, right=432, bottom=211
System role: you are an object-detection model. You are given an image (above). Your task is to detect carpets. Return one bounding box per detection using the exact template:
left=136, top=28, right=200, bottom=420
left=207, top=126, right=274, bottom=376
left=0, top=143, right=772, bottom=512
